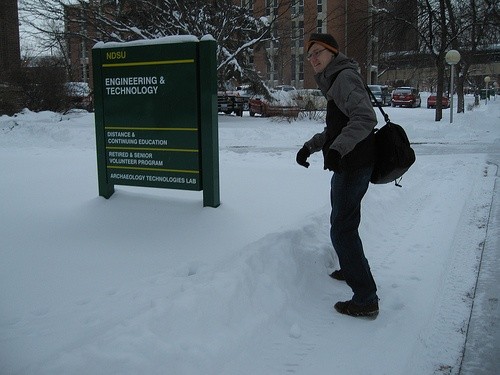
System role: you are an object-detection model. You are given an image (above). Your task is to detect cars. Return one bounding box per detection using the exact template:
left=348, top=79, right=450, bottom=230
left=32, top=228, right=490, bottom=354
left=427, top=90, right=450, bottom=109
left=217, top=82, right=328, bottom=111
left=66, top=81, right=93, bottom=110
left=248, top=88, right=300, bottom=118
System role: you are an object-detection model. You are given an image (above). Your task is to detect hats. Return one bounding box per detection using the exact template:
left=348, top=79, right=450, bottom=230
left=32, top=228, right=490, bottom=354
left=307, top=33, right=340, bottom=55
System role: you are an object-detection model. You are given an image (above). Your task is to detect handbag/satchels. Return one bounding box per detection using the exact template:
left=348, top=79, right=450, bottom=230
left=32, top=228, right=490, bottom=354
left=333, top=69, right=415, bottom=186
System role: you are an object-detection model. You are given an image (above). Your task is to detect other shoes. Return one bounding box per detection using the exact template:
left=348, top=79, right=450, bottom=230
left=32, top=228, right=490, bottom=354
left=335, top=301, right=379, bottom=316
left=330, top=270, right=345, bottom=280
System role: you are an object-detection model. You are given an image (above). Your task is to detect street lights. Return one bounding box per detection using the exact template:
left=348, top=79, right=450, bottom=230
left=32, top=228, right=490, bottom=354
left=493, top=81, right=498, bottom=100
left=484, top=75, right=491, bottom=105
left=446, top=49, right=461, bottom=123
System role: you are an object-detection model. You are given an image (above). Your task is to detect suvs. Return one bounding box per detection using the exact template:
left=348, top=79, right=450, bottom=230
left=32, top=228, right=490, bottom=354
left=367, top=84, right=392, bottom=107
left=390, top=86, right=421, bottom=108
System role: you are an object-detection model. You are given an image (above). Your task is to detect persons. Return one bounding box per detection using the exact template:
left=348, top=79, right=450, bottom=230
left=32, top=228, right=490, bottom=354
left=296, top=33, right=380, bottom=317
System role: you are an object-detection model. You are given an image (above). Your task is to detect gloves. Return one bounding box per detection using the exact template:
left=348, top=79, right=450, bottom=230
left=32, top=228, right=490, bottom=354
left=323, top=149, right=340, bottom=171
left=296, top=146, right=311, bottom=168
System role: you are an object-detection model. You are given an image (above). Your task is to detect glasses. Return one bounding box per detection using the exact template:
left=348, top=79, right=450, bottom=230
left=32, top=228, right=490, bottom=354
left=306, top=48, right=327, bottom=62
